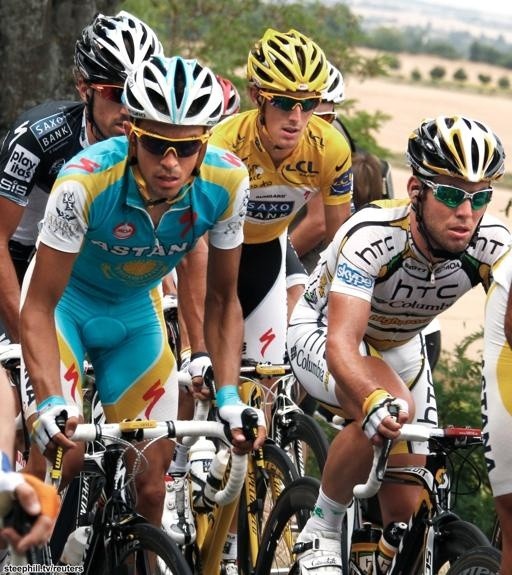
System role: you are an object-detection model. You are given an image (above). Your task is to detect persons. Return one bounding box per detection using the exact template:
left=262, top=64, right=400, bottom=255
left=2, top=11, right=510, bottom=574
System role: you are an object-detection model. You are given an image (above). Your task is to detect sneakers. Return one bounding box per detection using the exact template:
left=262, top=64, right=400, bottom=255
left=293, top=527, right=343, bottom=575
left=164, top=472, right=197, bottom=542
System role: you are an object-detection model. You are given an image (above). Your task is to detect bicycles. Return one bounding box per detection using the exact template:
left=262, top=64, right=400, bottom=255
left=1, top=361, right=502, bottom=575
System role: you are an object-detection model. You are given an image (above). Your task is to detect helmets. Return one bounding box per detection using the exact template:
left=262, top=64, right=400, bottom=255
left=71, top=10, right=346, bottom=128
left=405, top=113, right=506, bottom=182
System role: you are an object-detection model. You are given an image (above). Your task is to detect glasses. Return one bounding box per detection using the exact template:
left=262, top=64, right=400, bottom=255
left=419, top=175, right=495, bottom=212
left=127, top=122, right=210, bottom=157
